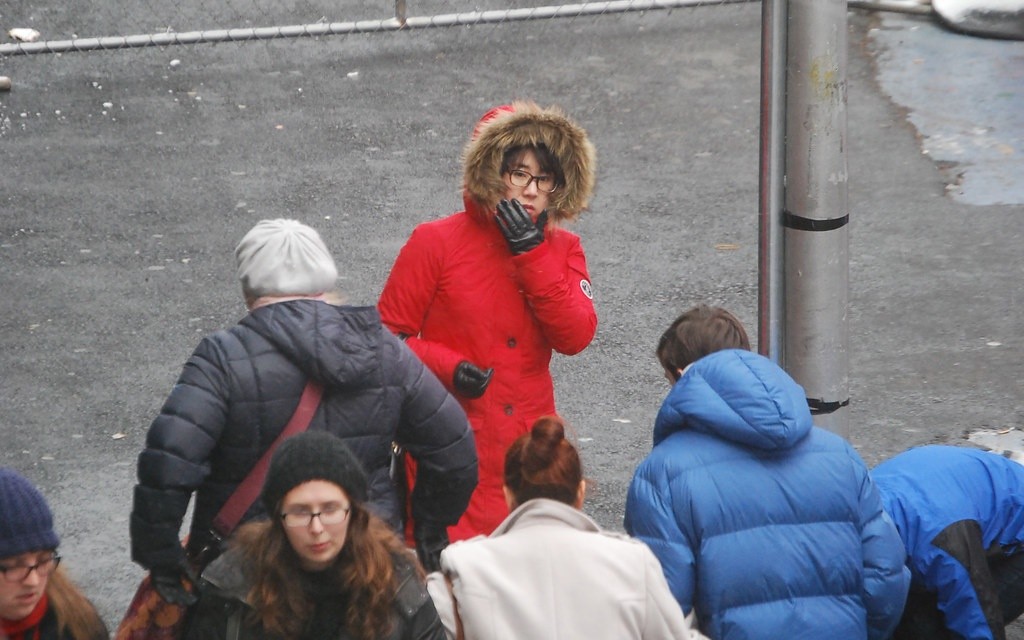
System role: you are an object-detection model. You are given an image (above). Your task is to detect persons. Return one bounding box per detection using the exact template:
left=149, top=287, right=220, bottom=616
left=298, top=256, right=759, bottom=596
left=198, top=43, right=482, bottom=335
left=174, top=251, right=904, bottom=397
left=131, top=217, right=478, bottom=603
left=0, top=469, right=107, bottom=640
left=378, top=99, right=598, bottom=548
left=173, top=431, right=451, bottom=640
left=623, top=305, right=911, bottom=640
left=425, top=418, right=710, bottom=640
left=867, top=444, right=1024, bottom=639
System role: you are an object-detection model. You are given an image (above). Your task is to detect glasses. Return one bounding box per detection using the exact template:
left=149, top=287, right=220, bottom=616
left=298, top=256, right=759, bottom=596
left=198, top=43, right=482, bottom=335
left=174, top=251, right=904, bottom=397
left=280, top=503, right=353, bottom=527
left=0, top=549, right=60, bottom=583
left=504, top=166, right=560, bottom=193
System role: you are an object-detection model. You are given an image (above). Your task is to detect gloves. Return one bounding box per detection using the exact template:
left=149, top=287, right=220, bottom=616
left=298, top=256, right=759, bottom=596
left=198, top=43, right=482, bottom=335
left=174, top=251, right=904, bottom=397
left=495, top=198, right=548, bottom=255
left=453, top=360, right=494, bottom=399
left=148, top=548, right=204, bottom=608
left=414, top=520, right=450, bottom=575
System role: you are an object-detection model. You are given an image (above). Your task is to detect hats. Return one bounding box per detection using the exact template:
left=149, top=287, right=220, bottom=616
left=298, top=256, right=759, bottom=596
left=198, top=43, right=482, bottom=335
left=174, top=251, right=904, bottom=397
left=233, top=219, right=337, bottom=292
left=265, top=429, right=366, bottom=513
left=0, top=466, right=61, bottom=558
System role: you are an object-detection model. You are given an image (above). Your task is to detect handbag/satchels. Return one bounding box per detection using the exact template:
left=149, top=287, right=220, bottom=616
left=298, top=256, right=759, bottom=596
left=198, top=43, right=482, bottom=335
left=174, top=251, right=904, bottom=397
left=114, top=532, right=196, bottom=640
